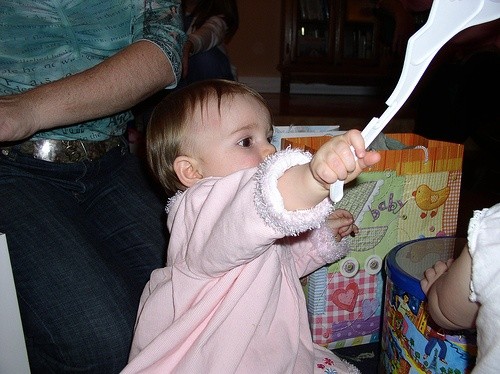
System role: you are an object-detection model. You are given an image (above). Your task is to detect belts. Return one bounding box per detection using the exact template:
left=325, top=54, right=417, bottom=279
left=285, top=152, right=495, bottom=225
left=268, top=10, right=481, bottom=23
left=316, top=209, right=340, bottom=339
left=0, top=132, right=129, bottom=164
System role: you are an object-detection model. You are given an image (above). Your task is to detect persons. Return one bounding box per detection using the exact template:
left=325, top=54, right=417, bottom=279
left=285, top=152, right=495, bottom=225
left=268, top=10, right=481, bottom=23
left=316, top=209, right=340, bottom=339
left=421, top=202, right=500, bottom=374
left=0, top=1, right=186, bottom=374
left=112, top=77, right=380, bottom=374
left=174, top=0, right=237, bottom=83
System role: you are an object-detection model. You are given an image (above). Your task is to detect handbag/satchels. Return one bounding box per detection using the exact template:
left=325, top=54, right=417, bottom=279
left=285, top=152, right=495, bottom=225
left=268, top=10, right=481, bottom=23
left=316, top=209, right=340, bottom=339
left=271, top=124, right=465, bottom=350
